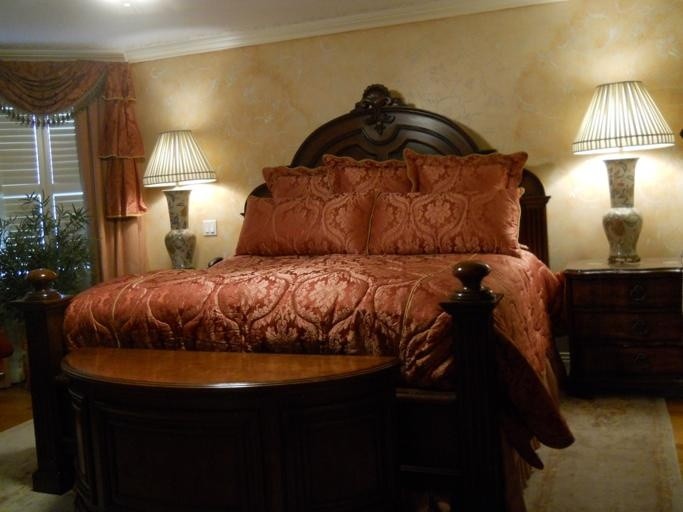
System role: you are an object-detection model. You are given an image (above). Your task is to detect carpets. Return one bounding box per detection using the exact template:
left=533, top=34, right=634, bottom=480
left=0, top=419, right=74, bottom=512
left=522, top=395, right=682, bottom=512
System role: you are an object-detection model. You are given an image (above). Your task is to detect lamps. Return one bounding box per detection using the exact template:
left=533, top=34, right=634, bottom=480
left=571, top=80, right=676, bottom=265
left=141, top=130, right=217, bottom=270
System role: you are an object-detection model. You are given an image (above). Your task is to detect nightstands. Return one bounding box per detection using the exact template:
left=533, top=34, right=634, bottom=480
left=561, top=256, right=682, bottom=398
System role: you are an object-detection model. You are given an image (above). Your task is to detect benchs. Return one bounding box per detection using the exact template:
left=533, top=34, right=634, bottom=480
left=60, top=348, right=403, bottom=511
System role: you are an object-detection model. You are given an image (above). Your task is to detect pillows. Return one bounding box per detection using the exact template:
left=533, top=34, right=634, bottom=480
left=234, top=147, right=529, bottom=260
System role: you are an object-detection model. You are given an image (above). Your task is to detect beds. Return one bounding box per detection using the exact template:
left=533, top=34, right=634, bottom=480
left=10, top=85, right=551, bottom=512
